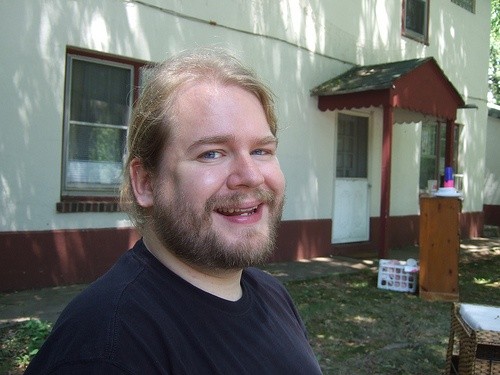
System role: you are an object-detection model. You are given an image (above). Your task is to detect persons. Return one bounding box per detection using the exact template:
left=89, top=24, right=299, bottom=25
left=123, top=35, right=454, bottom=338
left=25, top=44, right=325, bottom=375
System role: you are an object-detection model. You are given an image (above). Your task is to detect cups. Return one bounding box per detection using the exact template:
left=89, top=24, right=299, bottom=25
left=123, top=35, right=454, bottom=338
left=428, top=179, right=438, bottom=193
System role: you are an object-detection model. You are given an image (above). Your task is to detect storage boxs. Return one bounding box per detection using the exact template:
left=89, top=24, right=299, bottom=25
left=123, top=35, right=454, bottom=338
left=376, top=258, right=419, bottom=293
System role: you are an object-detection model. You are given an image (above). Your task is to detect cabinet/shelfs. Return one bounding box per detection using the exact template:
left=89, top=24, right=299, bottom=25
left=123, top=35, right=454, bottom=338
left=420, top=189, right=462, bottom=303
